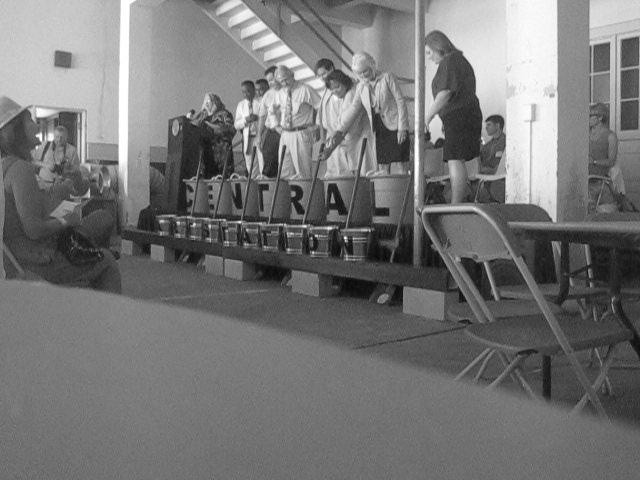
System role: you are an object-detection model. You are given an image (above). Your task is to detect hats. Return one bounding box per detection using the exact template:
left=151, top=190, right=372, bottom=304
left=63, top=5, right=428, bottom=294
left=0, top=96, right=33, bottom=129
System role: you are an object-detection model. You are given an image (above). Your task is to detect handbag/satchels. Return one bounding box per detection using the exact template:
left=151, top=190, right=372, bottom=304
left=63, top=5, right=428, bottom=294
left=57, top=226, right=104, bottom=266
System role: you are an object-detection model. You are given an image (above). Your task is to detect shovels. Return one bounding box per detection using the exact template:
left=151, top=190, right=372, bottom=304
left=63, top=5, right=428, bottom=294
left=178, top=148, right=230, bottom=270
left=370, top=152, right=416, bottom=306
left=280, top=143, right=324, bottom=288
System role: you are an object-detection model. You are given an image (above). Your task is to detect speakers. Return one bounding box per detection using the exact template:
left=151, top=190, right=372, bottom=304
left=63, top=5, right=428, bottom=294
left=54, top=50, right=72, bottom=68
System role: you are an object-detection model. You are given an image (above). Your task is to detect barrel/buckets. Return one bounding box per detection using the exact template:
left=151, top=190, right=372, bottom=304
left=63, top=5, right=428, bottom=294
left=154, top=173, right=414, bottom=261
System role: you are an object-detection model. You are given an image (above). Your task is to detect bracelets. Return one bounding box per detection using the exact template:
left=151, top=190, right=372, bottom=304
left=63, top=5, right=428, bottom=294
left=593, top=159, right=597, bottom=164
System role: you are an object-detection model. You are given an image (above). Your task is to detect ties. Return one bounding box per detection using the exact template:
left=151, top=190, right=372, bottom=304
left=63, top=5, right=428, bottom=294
left=284, top=90, right=292, bottom=131
left=248, top=101, right=256, bottom=136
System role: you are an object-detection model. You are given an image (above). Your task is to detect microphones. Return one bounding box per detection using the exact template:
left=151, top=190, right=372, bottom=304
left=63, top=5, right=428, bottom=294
left=188, top=109, right=196, bottom=115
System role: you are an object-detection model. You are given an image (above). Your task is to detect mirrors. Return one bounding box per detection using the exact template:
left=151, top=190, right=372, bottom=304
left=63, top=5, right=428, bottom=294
left=31, top=103, right=87, bottom=163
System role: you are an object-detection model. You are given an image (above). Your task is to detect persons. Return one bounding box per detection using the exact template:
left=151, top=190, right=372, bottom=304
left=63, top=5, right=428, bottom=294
left=31, top=125, right=80, bottom=191
left=0, top=95, right=122, bottom=294
left=588, top=103, right=618, bottom=204
left=423, top=30, right=484, bottom=203
left=191, top=50, right=410, bottom=180
left=480, top=115, right=506, bottom=175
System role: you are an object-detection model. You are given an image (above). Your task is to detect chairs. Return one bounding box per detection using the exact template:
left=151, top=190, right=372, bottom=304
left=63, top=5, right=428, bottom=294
left=425, top=138, right=626, bottom=212
left=2, top=244, right=96, bottom=291
